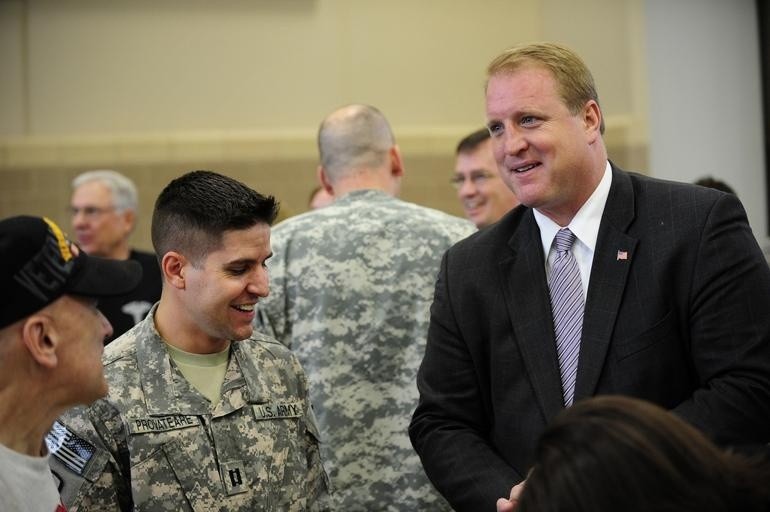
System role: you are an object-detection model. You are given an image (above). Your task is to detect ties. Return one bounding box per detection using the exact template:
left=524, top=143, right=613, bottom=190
left=550, top=229, right=585, bottom=407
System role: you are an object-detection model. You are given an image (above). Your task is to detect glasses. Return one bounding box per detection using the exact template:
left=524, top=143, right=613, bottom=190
left=450, top=174, right=501, bottom=187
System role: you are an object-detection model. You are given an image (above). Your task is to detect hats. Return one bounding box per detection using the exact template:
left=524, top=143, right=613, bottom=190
left=0, top=216, right=142, bottom=329
